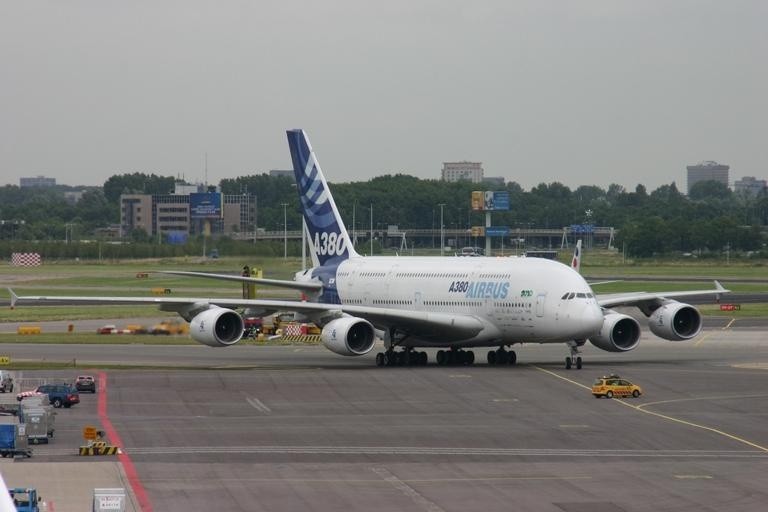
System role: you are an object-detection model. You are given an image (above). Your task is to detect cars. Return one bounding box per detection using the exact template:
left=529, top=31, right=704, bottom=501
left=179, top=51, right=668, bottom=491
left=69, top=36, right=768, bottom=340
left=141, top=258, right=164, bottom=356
left=76, top=375, right=95, bottom=393
left=16, top=383, right=80, bottom=408
left=591, top=373, right=643, bottom=398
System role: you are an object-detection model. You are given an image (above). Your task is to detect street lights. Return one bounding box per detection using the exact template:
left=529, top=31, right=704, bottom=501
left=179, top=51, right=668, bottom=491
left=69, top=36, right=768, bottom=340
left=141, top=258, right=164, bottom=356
left=279, top=203, right=289, bottom=257
left=352, top=198, right=359, bottom=246
left=438, top=203, right=448, bottom=255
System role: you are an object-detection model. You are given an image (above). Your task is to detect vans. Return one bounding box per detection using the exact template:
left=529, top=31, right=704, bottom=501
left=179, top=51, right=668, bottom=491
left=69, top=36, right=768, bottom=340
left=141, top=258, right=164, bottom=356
left=459, top=246, right=484, bottom=256
left=0, top=370, right=13, bottom=393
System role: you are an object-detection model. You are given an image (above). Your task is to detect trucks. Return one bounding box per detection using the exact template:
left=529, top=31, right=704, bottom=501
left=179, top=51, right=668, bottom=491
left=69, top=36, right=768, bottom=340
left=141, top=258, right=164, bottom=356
left=9, top=487, right=42, bottom=512
left=0, top=397, right=56, bottom=457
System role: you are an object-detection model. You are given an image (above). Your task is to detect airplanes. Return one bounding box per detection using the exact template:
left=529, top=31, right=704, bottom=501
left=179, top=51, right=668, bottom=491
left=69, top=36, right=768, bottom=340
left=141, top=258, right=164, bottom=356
left=7, top=128, right=733, bottom=370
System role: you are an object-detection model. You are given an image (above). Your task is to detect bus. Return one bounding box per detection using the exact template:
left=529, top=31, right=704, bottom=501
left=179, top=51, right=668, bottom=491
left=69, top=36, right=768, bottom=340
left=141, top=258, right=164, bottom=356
left=525, top=251, right=558, bottom=260
